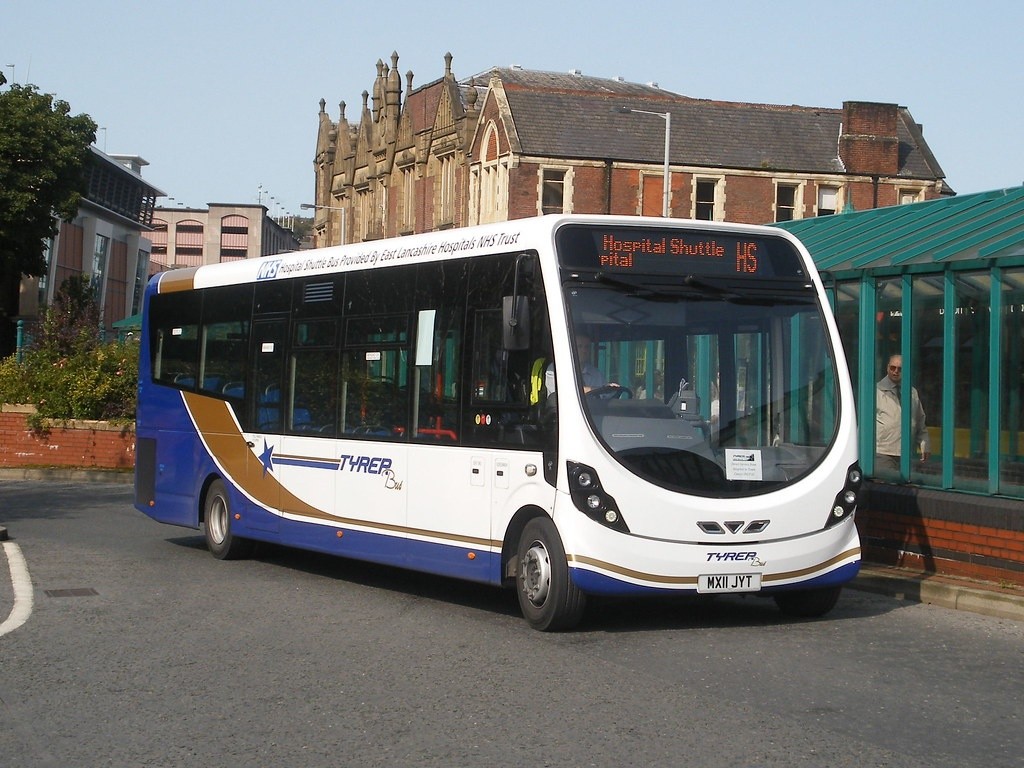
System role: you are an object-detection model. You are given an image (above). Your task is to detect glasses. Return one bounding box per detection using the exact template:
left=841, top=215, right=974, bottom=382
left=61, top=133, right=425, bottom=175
left=889, top=365, right=902, bottom=373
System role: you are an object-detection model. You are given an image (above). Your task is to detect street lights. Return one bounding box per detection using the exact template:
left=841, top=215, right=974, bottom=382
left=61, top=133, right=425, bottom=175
left=616, top=104, right=672, bottom=217
left=301, top=203, right=346, bottom=246
left=4, top=64, right=15, bottom=85
left=101, top=127, right=107, bottom=154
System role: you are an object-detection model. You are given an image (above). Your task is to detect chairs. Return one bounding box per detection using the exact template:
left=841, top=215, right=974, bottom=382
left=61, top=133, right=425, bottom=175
left=173, top=367, right=435, bottom=440
left=529, top=357, right=549, bottom=425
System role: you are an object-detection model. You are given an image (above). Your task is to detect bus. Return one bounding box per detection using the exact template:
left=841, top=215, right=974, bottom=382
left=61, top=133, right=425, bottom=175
left=133, top=213, right=866, bottom=632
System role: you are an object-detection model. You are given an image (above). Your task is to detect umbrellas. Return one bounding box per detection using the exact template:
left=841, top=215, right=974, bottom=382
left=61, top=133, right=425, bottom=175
left=111, top=313, right=143, bottom=328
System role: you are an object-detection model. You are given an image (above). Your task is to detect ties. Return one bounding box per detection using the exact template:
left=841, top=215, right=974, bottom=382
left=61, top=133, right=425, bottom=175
left=896, top=386, right=901, bottom=407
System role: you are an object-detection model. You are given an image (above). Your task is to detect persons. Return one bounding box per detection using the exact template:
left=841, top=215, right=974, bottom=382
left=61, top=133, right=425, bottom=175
left=876, top=354, right=932, bottom=471
left=544, top=331, right=619, bottom=399
left=636, top=369, right=662, bottom=399
left=711, top=382, right=719, bottom=446
